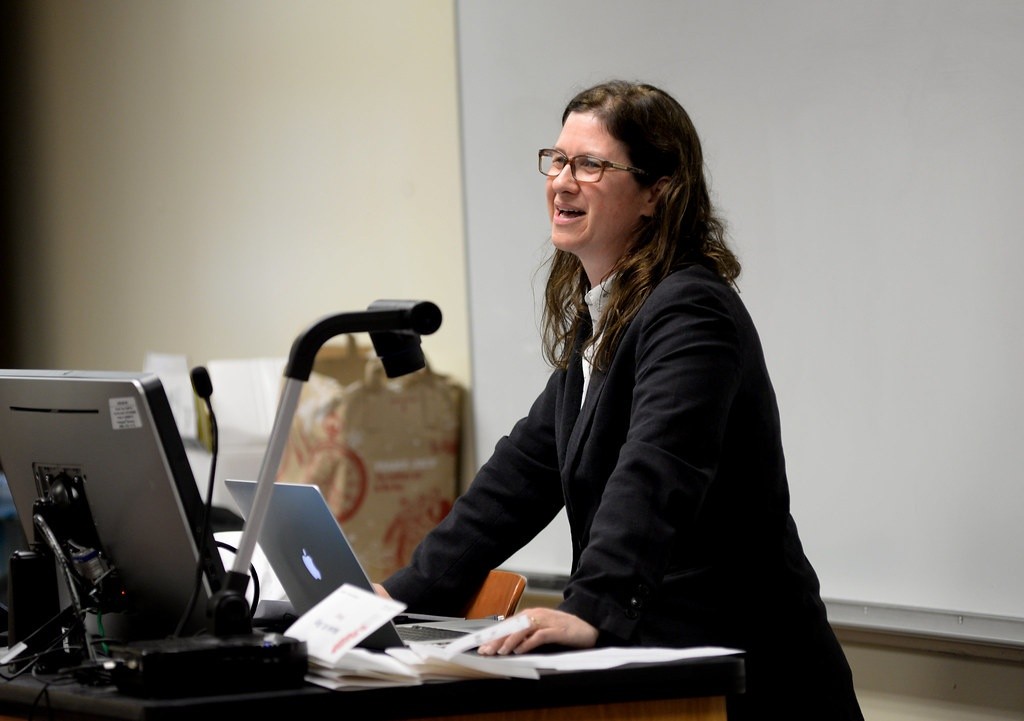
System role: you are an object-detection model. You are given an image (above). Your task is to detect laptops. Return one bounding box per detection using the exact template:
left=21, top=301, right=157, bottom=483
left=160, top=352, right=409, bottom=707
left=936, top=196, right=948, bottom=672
left=223, top=478, right=503, bottom=655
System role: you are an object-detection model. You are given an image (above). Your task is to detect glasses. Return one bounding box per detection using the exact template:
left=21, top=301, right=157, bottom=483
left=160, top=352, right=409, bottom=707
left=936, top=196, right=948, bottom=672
left=538, top=148, right=658, bottom=183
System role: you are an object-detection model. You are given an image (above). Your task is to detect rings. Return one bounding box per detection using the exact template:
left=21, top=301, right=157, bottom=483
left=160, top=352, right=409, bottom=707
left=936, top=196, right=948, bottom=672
left=531, top=617, right=541, bottom=626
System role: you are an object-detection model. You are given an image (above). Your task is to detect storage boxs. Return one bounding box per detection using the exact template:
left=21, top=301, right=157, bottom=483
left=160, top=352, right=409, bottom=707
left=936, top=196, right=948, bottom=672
left=278, top=334, right=463, bottom=586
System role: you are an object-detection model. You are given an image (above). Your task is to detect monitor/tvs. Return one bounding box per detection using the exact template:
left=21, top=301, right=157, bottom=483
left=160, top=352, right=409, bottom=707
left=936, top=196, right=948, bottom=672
left=0, top=369, right=230, bottom=678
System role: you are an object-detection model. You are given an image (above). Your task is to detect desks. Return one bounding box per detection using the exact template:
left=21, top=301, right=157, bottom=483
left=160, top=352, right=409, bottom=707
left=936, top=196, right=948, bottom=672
left=0, top=635, right=746, bottom=721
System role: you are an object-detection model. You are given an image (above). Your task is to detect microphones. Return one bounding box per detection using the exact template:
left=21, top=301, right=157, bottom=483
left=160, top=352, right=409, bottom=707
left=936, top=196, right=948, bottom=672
left=189, top=367, right=220, bottom=524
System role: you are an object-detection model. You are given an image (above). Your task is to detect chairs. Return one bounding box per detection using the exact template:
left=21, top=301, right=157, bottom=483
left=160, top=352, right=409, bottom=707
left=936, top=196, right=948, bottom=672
left=465, top=570, right=528, bottom=620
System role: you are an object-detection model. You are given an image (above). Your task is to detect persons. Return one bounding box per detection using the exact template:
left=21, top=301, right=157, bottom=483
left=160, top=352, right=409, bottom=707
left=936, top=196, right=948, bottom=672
left=365, top=81, right=870, bottom=721
